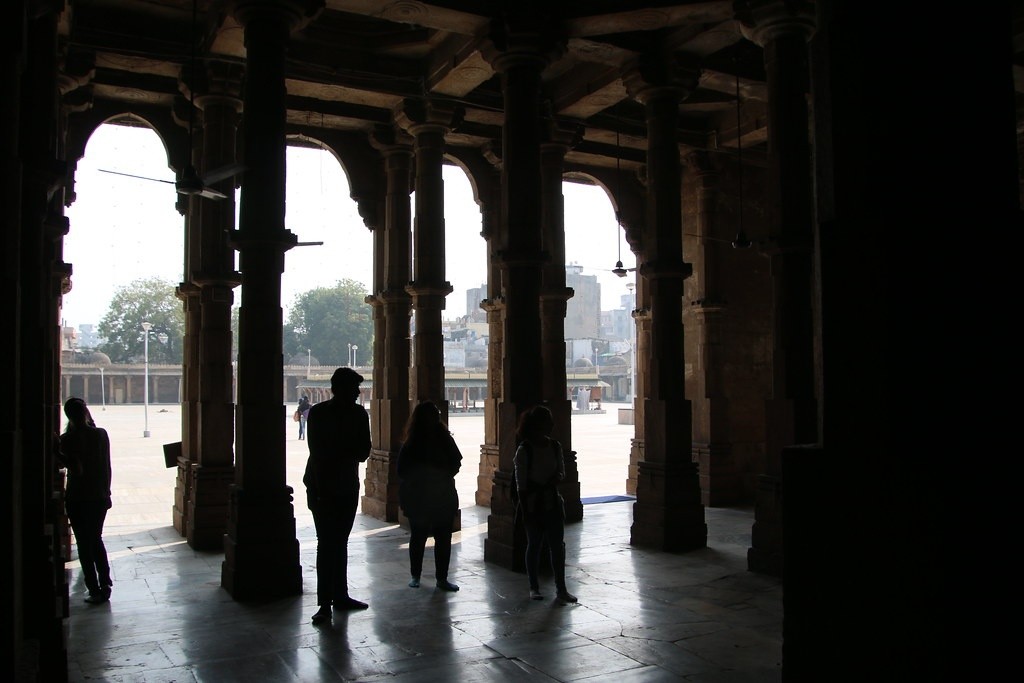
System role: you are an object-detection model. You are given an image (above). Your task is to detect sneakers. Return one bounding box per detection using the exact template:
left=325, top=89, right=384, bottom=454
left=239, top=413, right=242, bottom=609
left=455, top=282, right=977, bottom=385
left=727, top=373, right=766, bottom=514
left=334, top=597, right=368, bottom=611
left=436, top=580, right=459, bottom=592
left=311, top=608, right=332, bottom=622
left=408, top=577, right=421, bottom=587
left=557, top=592, right=577, bottom=603
left=530, top=589, right=543, bottom=600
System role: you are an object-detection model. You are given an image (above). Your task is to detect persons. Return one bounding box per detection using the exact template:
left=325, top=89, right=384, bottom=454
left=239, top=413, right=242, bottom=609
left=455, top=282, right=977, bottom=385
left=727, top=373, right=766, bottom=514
left=296, top=396, right=312, bottom=440
left=396, top=401, right=463, bottom=592
left=302, top=368, right=375, bottom=623
left=55, top=396, right=113, bottom=606
left=511, top=406, right=578, bottom=604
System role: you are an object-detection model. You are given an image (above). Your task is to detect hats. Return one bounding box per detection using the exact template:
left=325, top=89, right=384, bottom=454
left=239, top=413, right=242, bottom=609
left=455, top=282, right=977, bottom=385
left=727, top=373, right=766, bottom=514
left=331, top=367, right=364, bottom=385
